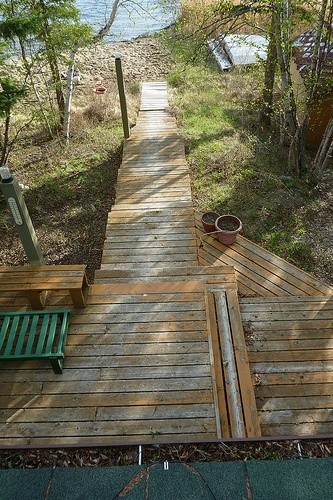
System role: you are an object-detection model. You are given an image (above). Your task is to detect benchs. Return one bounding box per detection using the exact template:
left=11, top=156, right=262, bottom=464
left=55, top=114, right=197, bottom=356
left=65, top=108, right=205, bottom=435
left=0, top=264, right=90, bottom=310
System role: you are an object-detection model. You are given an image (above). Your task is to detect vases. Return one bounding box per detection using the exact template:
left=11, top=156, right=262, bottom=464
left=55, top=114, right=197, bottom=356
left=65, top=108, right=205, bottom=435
left=201, top=211, right=220, bottom=232
left=214, top=215, right=243, bottom=246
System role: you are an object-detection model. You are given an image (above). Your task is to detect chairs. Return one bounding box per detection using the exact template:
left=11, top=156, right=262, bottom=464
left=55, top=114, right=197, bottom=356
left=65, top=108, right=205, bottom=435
left=0, top=309, right=72, bottom=374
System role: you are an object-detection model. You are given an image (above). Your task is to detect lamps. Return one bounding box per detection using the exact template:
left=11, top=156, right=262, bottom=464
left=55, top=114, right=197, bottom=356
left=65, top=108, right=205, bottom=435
left=0, top=165, right=13, bottom=183
left=115, top=52, right=121, bottom=61
